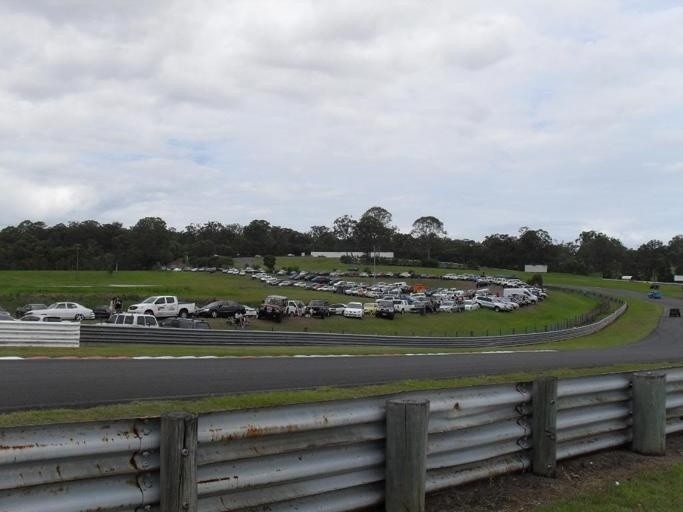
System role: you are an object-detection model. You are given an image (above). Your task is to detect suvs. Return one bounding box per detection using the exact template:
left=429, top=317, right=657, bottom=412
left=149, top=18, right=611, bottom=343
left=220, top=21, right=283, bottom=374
left=670, top=309, right=680, bottom=318
left=650, top=283, right=659, bottom=289
left=108, top=312, right=210, bottom=329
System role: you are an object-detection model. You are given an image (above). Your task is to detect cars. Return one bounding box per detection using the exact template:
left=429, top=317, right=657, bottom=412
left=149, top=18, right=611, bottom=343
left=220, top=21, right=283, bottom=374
left=196, top=300, right=259, bottom=320
left=14, top=301, right=113, bottom=321
left=252, top=267, right=550, bottom=321
left=161, top=264, right=255, bottom=277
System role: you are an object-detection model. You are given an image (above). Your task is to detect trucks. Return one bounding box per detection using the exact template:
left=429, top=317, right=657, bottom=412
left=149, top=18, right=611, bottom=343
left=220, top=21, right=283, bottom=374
left=648, top=290, right=661, bottom=299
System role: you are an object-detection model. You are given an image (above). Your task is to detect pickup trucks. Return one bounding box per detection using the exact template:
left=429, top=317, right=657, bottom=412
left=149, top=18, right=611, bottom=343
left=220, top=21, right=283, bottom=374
left=127, top=296, right=195, bottom=318
